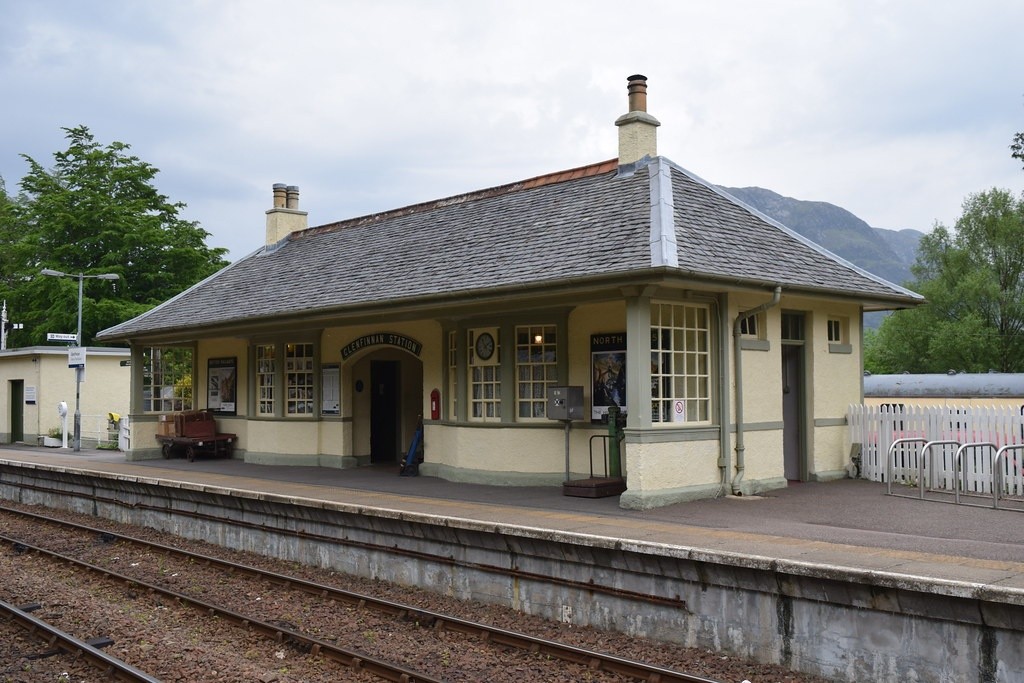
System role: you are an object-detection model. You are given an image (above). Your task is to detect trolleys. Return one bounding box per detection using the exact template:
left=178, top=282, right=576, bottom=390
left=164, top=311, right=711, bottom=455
left=399, top=415, right=425, bottom=477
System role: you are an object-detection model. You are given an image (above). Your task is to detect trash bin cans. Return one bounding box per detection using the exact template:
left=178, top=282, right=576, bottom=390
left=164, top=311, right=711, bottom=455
left=106, top=412, right=120, bottom=447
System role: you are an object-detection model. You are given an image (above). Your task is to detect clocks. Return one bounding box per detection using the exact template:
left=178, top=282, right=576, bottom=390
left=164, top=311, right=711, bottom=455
left=475, top=332, right=495, bottom=362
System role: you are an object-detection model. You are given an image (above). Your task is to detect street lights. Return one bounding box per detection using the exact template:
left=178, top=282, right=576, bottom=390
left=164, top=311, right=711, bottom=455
left=40, top=267, right=120, bottom=453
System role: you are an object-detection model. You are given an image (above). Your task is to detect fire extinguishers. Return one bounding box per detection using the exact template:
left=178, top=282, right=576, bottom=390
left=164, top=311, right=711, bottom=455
left=430, top=387, right=441, bottom=420
